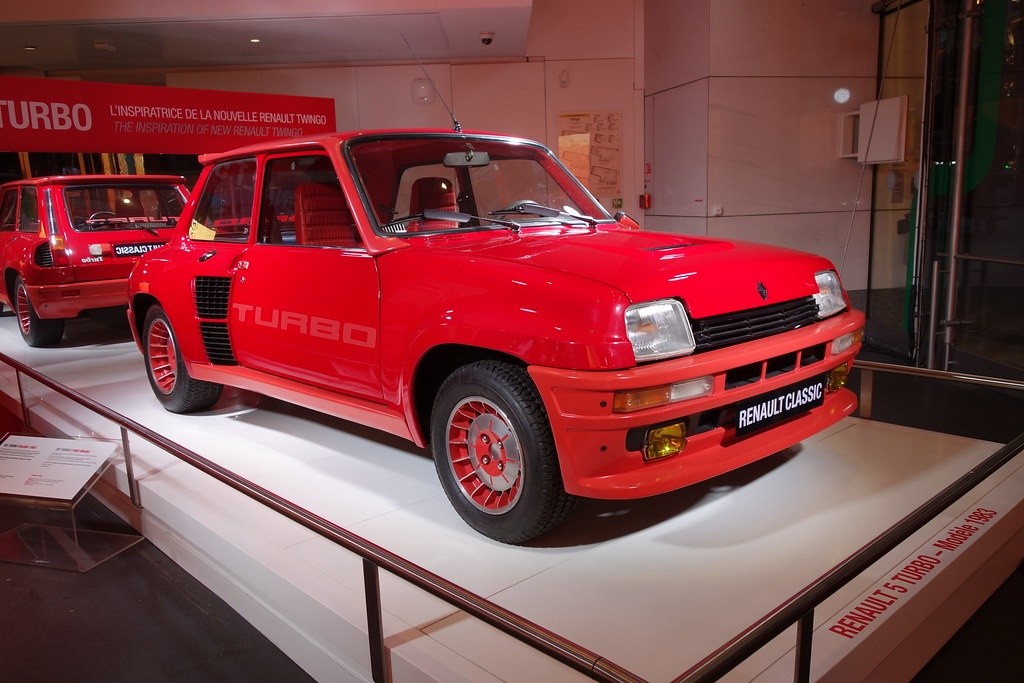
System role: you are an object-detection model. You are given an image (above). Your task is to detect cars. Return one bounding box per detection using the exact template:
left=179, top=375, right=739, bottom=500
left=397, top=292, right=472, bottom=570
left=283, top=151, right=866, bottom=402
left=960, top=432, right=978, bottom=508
left=0, top=174, right=214, bottom=347
left=126, top=128, right=864, bottom=539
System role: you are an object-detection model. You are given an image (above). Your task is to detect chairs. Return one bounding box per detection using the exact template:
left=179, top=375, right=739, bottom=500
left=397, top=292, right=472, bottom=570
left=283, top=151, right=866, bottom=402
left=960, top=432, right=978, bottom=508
left=294, top=180, right=356, bottom=248
left=406, top=177, right=459, bottom=233
